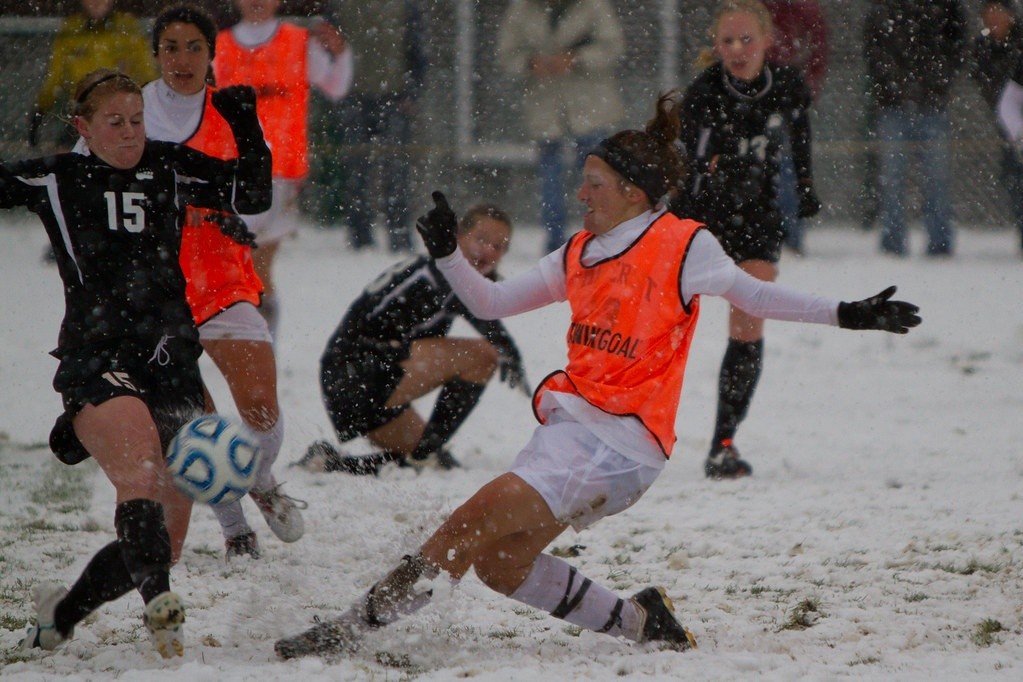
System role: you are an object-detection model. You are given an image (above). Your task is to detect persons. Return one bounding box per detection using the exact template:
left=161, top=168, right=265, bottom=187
left=0, top=70, right=273, bottom=660
left=860, top=0, right=971, bottom=254
left=971, top=0, right=1023, bottom=247
left=677, top=0, right=823, bottom=476
left=30, top=0, right=157, bottom=264
left=764, top=0, right=826, bottom=252
left=272, top=85, right=922, bottom=662
left=325, top=0, right=430, bottom=256
left=71, top=1, right=302, bottom=565
left=494, top=0, right=625, bottom=250
left=208, top=0, right=351, bottom=340
left=285, top=205, right=532, bottom=476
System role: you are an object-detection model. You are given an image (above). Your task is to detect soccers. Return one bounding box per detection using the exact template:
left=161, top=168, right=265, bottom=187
left=165, top=414, right=264, bottom=506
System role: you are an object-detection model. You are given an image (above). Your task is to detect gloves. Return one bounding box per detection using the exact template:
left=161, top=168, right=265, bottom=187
left=204, top=213, right=258, bottom=249
left=500, top=347, right=533, bottom=398
left=719, top=153, right=765, bottom=180
left=796, top=182, right=820, bottom=220
left=210, top=84, right=259, bottom=130
left=415, top=191, right=458, bottom=258
left=837, top=285, right=922, bottom=334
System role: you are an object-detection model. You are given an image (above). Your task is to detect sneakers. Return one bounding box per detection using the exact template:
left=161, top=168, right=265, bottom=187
left=248, top=474, right=309, bottom=543
left=274, top=619, right=360, bottom=662
left=142, top=591, right=186, bottom=661
left=285, top=439, right=339, bottom=473
left=225, top=532, right=261, bottom=569
left=705, top=440, right=753, bottom=479
left=629, top=585, right=700, bottom=653
left=9, top=581, right=74, bottom=664
